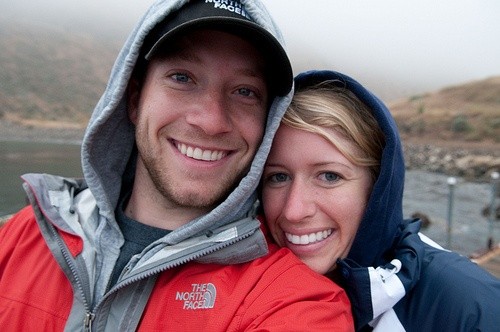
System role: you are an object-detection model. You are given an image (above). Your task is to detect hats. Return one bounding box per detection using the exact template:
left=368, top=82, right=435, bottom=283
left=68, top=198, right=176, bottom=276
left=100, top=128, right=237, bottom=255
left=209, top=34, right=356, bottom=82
left=142, top=0, right=293, bottom=97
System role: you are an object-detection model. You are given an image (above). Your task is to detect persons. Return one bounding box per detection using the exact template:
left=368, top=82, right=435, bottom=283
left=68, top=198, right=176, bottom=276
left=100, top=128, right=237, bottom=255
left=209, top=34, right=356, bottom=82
left=258, top=70, right=500, bottom=332
left=0, top=0, right=356, bottom=332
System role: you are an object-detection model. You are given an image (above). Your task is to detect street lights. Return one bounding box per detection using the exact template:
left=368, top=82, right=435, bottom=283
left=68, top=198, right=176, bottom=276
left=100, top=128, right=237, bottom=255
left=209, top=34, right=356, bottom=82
left=446, top=176, right=457, bottom=250
left=488, top=172, right=500, bottom=249
